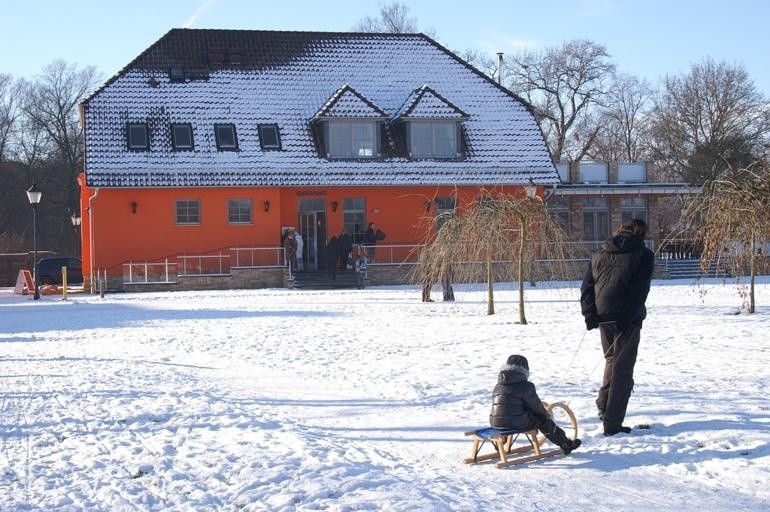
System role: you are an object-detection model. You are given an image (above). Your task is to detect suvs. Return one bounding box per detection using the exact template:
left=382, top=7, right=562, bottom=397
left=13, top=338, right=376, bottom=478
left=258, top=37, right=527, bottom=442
left=33, top=256, right=83, bottom=287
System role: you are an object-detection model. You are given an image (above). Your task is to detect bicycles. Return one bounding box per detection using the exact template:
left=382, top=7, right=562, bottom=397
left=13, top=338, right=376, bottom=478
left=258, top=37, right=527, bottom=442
left=350, top=256, right=369, bottom=289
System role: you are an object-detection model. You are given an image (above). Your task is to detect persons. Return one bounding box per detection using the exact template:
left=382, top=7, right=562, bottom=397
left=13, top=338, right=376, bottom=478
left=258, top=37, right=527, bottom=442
left=352, top=224, right=364, bottom=243
left=326, top=237, right=339, bottom=280
left=581, top=220, right=653, bottom=436
left=284, top=231, right=298, bottom=269
left=293, top=231, right=304, bottom=271
left=489, top=354, right=581, bottom=456
left=365, top=222, right=376, bottom=261
left=338, top=230, right=353, bottom=272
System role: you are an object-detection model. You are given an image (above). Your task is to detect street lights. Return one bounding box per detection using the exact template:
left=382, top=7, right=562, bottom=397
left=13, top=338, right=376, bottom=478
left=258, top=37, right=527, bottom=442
left=525, top=176, right=537, bottom=285
left=25, top=183, right=44, bottom=300
left=69, top=211, right=82, bottom=256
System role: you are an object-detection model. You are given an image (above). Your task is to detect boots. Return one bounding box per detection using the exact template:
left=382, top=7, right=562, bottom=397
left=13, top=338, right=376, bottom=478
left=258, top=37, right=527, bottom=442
left=560, top=439, right=582, bottom=455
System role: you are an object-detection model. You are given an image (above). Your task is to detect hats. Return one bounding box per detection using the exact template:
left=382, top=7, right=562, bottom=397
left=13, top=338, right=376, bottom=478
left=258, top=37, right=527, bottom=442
left=506, top=355, right=528, bottom=371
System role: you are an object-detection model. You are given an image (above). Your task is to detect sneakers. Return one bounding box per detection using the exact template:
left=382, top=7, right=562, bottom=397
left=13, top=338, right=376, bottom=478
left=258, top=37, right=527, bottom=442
left=604, top=427, right=631, bottom=436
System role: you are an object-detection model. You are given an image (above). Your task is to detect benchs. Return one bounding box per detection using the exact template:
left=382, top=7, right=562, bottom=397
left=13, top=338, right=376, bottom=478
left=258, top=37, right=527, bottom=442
left=660, top=239, right=704, bottom=260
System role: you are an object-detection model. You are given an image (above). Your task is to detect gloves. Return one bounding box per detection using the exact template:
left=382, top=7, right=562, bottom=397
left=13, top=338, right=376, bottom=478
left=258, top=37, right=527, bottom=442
left=586, top=316, right=599, bottom=330
left=617, top=320, right=632, bottom=328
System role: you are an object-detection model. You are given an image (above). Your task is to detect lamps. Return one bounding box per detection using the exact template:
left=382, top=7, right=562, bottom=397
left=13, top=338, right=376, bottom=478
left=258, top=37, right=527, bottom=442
left=425, top=201, right=431, bottom=212
left=131, top=201, right=137, bottom=213
left=264, top=200, right=269, bottom=212
left=332, top=200, right=337, bottom=212
